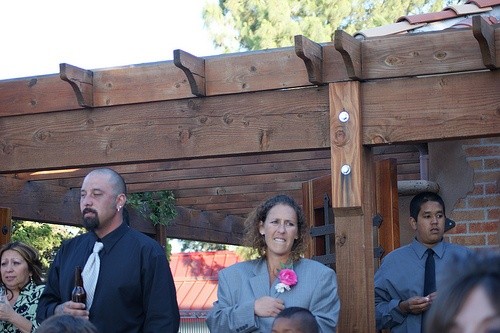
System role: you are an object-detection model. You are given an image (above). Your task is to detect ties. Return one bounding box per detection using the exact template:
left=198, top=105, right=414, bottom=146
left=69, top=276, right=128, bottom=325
left=424, top=249, right=436, bottom=303
left=78, top=241, right=104, bottom=313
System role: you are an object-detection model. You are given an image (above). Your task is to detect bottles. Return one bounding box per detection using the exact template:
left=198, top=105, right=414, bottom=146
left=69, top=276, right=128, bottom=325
left=72, top=264, right=87, bottom=310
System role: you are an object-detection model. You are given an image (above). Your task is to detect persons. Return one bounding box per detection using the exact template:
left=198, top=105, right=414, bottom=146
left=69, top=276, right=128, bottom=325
left=0, top=241, right=47, bottom=333
left=425, top=250, right=500, bottom=333
left=373, top=190, right=476, bottom=333
left=205, top=194, right=341, bottom=333
left=271, top=307, right=318, bottom=333
left=36, top=170, right=181, bottom=333
left=35, top=315, right=100, bottom=333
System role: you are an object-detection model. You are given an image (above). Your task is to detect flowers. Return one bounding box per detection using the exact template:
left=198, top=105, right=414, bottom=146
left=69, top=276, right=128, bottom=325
left=272, top=257, right=298, bottom=295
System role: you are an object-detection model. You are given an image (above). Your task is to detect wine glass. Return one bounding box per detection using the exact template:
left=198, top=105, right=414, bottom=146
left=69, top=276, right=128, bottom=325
left=0, top=286, right=8, bottom=324
